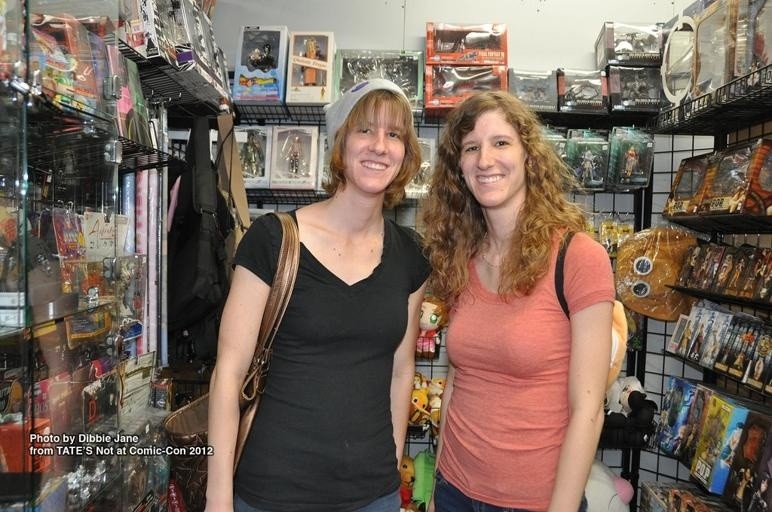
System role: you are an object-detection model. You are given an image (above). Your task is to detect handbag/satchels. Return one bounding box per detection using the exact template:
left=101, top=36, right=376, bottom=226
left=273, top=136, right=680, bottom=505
left=146, top=111, right=251, bottom=331
left=163, top=349, right=274, bottom=512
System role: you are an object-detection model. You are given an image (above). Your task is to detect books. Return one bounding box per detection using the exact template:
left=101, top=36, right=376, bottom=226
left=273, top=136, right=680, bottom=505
left=639, top=0, right=772, bottom=512
left=230, top=20, right=636, bottom=257
left=0, top=2, right=228, bottom=511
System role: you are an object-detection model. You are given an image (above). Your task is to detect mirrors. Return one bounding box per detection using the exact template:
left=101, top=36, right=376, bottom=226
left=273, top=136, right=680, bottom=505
left=662, top=2, right=768, bottom=106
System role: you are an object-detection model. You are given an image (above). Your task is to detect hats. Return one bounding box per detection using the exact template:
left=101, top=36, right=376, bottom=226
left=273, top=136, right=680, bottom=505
left=323, top=79, right=413, bottom=156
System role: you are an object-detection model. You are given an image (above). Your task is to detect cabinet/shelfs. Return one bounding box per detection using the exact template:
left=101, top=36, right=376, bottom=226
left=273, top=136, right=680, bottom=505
left=637, top=63, right=771, bottom=512
left=1, top=2, right=216, bottom=509
left=221, top=68, right=637, bottom=509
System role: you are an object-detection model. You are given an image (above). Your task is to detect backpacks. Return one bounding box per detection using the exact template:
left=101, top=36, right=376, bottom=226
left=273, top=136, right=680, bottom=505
left=555, top=230, right=628, bottom=391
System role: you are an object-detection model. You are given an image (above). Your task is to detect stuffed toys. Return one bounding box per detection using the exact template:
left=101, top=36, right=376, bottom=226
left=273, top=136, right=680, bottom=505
left=399, top=293, right=657, bottom=511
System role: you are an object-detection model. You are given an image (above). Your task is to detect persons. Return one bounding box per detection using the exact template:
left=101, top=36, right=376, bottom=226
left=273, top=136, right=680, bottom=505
left=639, top=0, right=772, bottom=512
left=0, top=2, right=228, bottom=511
left=399, top=293, right=657, bottom=511
left=230, top=20, right=636, bottom=257
left=203, top=77, right=437, bottom=512
left=413, top=89, right=616, bottom=510
